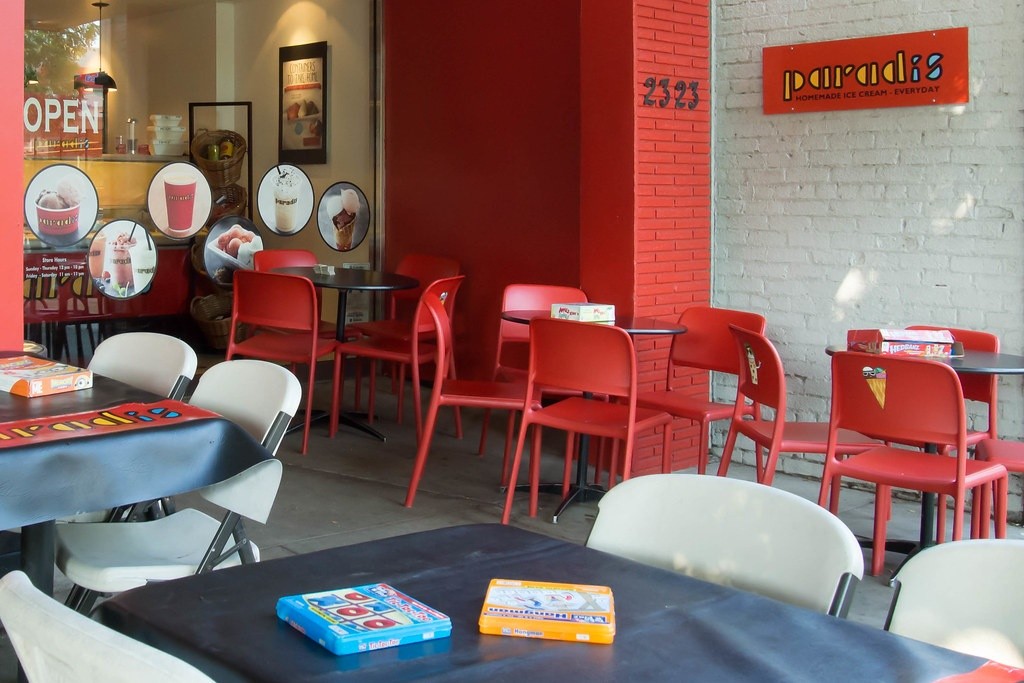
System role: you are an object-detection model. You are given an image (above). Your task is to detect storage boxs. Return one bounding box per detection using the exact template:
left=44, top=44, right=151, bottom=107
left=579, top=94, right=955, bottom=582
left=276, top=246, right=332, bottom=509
left=853, top=329, right=964, bottom=358
left=276, top=583, right=451, bottom=655
left=0, top=356, right=93, bottom=398
left=478, top=579, right=616, bottom=644
left=552, top=303, right=615, bottom=322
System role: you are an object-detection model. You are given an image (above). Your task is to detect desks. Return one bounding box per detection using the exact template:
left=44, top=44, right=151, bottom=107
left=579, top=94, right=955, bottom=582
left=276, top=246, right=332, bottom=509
left=0, top=349, right=286, bottom=681
left=500, top=309, right=689, bottom=525
left=266, top=264, right=421, bottom=442
left=87, top=525, right=1024, bottom=683
left=825, top=344, right=1023, bottom=588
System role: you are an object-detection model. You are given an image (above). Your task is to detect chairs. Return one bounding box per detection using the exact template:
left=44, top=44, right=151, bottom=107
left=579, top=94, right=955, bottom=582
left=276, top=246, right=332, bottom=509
left=0, top=247, right=1024, bottom=683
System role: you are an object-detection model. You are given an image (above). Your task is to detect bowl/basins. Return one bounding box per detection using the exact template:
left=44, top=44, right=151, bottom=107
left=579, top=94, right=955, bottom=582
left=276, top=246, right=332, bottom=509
left=153, top=140, right=185, bottom=156
left=35, top=201, right=80, bottom=234
left=150, top=113, right=182, bottom=126
left=154, top=126, right=186, bottom=142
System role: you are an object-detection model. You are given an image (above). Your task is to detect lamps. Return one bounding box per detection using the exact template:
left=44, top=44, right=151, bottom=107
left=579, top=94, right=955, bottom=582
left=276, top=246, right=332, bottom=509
left=75, top=3, right=118, bottom=92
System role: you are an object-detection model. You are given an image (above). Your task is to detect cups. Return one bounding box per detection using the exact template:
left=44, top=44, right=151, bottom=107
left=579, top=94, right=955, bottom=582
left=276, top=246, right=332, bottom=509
left=89, top=236, right=134, bottom=288
left=163, top=177, right=196, bottom=230
left=331, top=210, right=357, bottom=250
left=271, top=174, right=302, bottom=232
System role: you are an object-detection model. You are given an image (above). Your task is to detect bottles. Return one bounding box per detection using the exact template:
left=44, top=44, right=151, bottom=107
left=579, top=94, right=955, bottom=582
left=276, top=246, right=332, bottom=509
left=115, top=118, right=150, bottom=155
left=207, top=138, right=233, bottom=161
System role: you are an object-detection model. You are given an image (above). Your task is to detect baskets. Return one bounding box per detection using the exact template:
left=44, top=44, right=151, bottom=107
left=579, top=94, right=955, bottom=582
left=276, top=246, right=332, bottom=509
left=190, top=128, right=247, bottom=188
left=191, top=243, right=208, bottom=276
left=190, top=291, right=245, bottom=349
left=205, top=183, right=247, bottom=231
